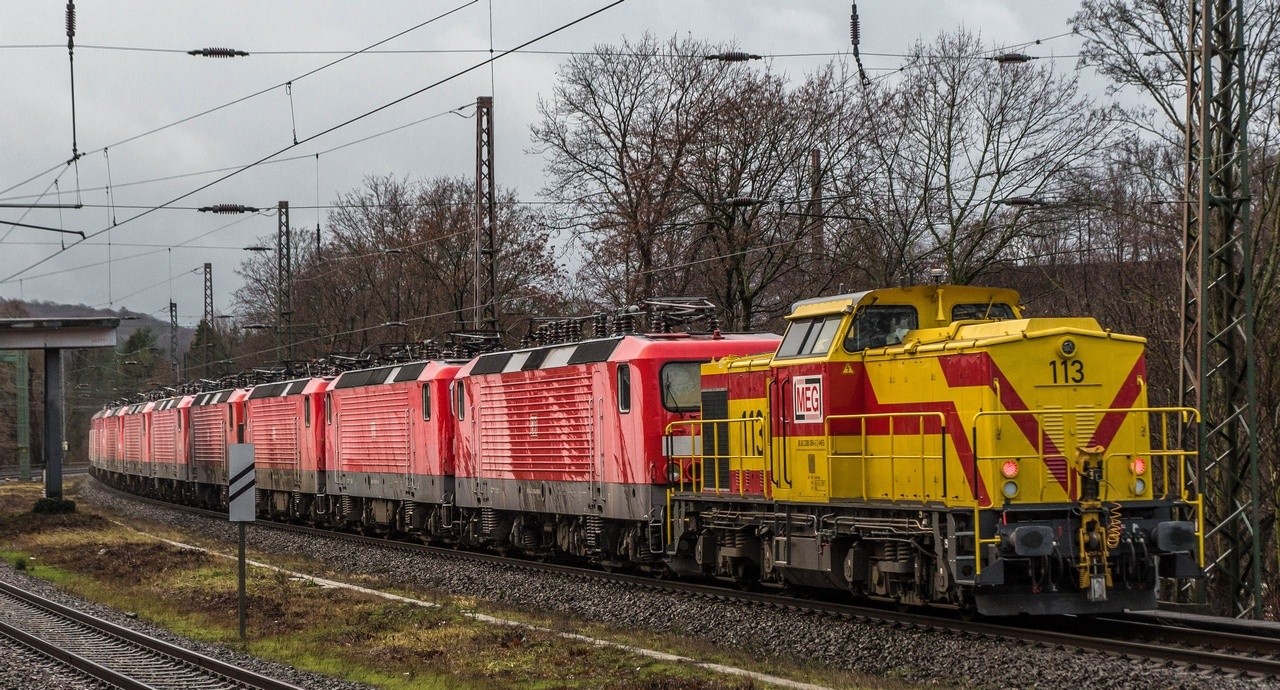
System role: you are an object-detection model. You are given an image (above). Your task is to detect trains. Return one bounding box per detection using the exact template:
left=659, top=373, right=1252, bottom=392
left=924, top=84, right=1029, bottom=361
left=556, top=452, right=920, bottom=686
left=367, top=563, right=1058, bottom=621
left=87, top=260, right=1208, bottom=629
